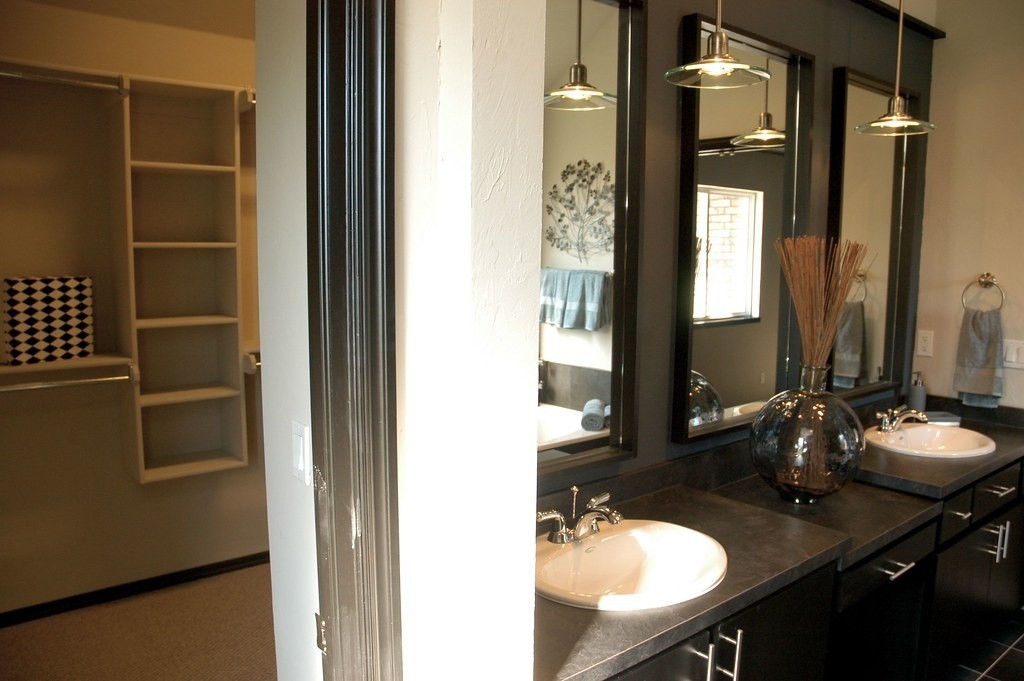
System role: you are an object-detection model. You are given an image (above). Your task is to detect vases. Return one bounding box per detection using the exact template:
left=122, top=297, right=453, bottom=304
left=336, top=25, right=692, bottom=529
left=748, top=364, right=866, bottom=505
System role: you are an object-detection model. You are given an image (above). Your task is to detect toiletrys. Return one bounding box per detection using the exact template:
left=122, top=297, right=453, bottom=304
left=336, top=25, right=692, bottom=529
left=908, top=370, right=927, bottom=412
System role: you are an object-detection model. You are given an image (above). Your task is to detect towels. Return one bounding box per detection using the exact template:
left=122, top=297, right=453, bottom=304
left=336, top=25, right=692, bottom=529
left=951, top=306, right=1004, bottom=409
left=539, top=267, right=611, bottom=333
left=832, top=300, right=868, bottom=389
left=580, top=399, right=610, bottom=432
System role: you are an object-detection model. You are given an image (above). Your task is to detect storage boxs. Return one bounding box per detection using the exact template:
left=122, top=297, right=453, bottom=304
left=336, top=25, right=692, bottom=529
left=2, top=275, right=95, bottom=365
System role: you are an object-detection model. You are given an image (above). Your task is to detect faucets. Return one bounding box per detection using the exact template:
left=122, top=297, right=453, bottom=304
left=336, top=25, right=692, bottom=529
left=875, top=404, right=928, bottom=434
left=536, top=485, right=624, bottom=544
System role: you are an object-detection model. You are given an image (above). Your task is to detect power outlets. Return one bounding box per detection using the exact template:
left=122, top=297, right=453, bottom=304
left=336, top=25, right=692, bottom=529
left=917, top=330, right=934, bottom=357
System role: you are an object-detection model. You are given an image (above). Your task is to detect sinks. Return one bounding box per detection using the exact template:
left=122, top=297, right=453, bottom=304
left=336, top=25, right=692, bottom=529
left=864, top=423, right=996, bottom=459
left=535, top=519, right=728, bottom=612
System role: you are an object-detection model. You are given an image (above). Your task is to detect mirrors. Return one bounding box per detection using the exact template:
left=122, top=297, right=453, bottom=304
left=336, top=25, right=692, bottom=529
left=824, top=66, right=920, bottom=400
left=537, top=0, right=647, bottom=479
left=671, top=11, right=817, bottom=446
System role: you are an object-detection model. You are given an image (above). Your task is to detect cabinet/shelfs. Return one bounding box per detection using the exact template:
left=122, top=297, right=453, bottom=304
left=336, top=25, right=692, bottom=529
left=1, top=54, right=259, bottom=483
left=836, top=518, right=940, bottom=680
left=604, top=559, right=837, bottom=681
left=929, top=460, right=1023, bottom=680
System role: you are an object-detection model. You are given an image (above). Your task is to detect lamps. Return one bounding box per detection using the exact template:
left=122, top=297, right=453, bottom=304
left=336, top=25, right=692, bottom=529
left=853, top=0, right=936, bottom=135
left=731, top=56, right=786, bottom=149
left=663, top=0, right=772, bottom=90
left=544, top=0, right=626, bottom=110
